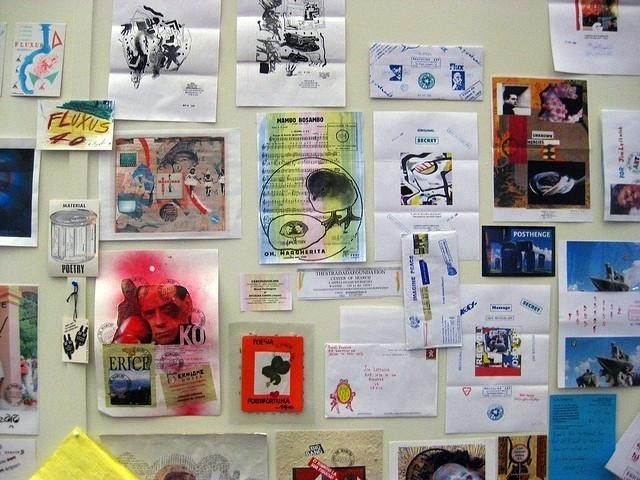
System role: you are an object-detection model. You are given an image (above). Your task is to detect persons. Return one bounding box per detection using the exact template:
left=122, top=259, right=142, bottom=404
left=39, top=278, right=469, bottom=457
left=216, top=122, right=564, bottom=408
left=217, top=166, right=225, bottom=195
left=611, top=183, right=639, bottom=214
left=111, top=283, right=206, bottom=345
left=185, top=166, right=202, bottom=199
left=389, top=65, right=402, bottom=80
left=502, top=89, right=520, bottom=116
left=20, top=355, right=29, bottom=392
left=452, top=72, right=463, bottom=91
left=31, top=356, right=37, bottom=391
left=172, top=150, right=200, bottom=175
left=203, top=169, right=215, bottom=196
